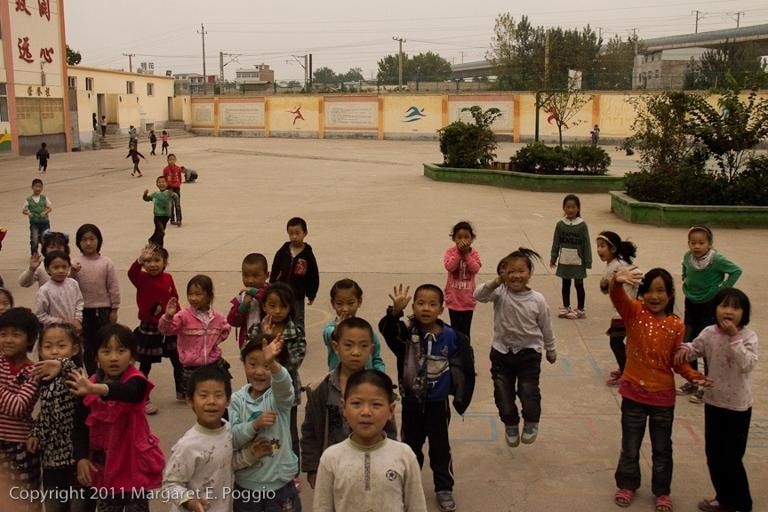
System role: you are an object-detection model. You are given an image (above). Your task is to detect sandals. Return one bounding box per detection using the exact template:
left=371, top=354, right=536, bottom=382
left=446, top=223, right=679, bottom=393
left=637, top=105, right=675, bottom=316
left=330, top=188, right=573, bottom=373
left=655, top=496, right=672, bottom=512
left=615, top=488, right=634, bottom=507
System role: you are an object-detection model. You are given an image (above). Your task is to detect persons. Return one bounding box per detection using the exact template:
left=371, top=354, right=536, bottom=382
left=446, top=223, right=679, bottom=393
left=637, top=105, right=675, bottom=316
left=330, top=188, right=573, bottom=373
left=100, top=115, right=108, bottom=138
left=1, top=193, right=767, bottom=512
left=162, top=154, right=182, bottom=227
left=149, top=130, right=157, bottom=156
left=160, top=130, right=170, bottom=155
left=22, top=178, right=52, bottom=255
left=128, top=125, right=135, bottom=147
left=92, top=113, right=97, bottom=130
left=143, top=176, right=179, bottom=246
left=590, top=124, right=600, bottom=146
left=179, top=166, right=198, bottom=183
left=123, top=143, right=147, bottom=178
left=36, top=143, right=50, bottom=173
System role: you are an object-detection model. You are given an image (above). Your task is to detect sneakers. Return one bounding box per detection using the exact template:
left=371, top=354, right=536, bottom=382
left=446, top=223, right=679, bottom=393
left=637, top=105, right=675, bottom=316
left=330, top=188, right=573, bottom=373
left=698, top=499, right=724, bottom=511
left=521, top=422, right=537, bottom=443
left=144, top=401, right=158, bottom=415
left=505, top=425, right=520, bottom=447
left=676, top=382, right=698, bottom=395
left=435, top=491, right=456, bottom=512
left=559, top=306, right=569, bottom=318
left=690, top=391, right=704, bottom=403
left=607, top=377, right=621, bottom=386
left=568, top=309, right=585, bottom=319
left=610, top=369, right=622, bottom=377
